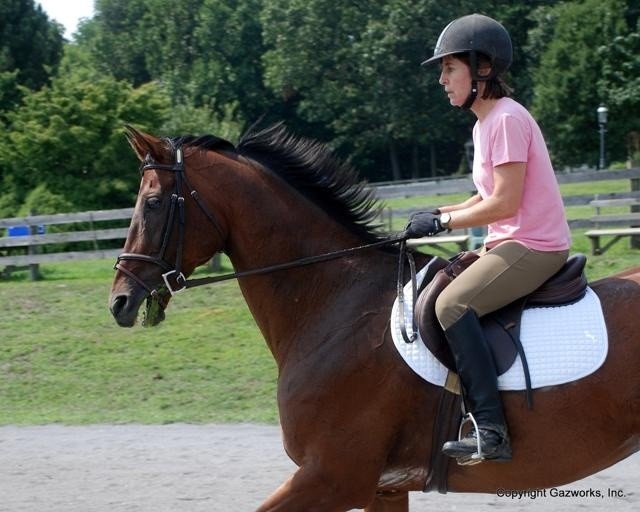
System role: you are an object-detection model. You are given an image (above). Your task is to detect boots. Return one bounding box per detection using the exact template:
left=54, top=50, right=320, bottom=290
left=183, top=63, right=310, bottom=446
left=439, top=307, right=516, bottom=462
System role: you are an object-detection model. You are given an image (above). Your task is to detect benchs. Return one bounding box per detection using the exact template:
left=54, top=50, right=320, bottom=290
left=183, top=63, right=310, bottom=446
left=584, top=196, right=639, bottom=255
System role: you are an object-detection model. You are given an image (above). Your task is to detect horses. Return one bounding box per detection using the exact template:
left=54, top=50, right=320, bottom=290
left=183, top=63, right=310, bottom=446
left=106, top=118, right=640, bottom=512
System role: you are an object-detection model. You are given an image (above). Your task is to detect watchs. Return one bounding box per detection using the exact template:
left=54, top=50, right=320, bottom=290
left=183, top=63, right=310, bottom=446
left=440, top=212, right=452, bottom=229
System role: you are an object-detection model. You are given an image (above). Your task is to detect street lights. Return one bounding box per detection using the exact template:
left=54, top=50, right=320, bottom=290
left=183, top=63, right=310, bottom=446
left=596, top=102, right=609, bottom=170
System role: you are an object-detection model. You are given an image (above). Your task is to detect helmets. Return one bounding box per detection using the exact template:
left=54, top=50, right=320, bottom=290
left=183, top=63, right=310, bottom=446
left=419, top=12, right=514, bottom=73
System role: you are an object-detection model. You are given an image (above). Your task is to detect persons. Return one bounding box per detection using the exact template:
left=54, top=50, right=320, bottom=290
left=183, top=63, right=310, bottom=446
left=400, top=14, right=571, bottom=462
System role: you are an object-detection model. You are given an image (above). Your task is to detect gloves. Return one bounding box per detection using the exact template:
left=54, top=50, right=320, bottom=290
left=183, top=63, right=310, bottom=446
left=403, top=208, right=445, bottom=238
